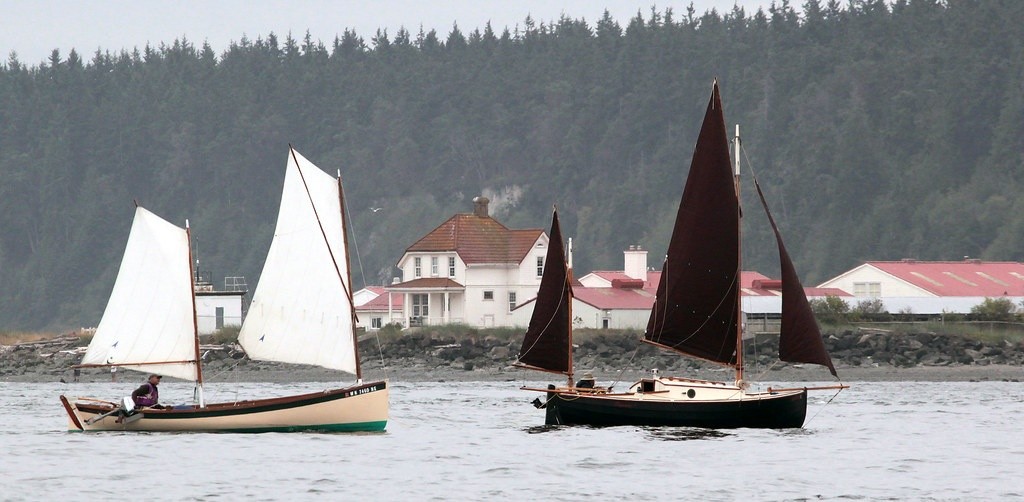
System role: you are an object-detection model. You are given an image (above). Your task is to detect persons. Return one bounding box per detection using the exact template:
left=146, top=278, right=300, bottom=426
left=132, top=374, right=164, bottom=409
left=576, top=373, right=594, bottom=388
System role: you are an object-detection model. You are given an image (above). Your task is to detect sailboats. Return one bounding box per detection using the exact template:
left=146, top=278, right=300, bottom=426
left=60, top=143, right=391, bottom=433
left=510, top=77, right=850, bottom=430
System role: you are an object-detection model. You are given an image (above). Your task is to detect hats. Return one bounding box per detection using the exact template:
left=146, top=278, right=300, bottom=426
left=148, top=374, right=162, bottom=380
